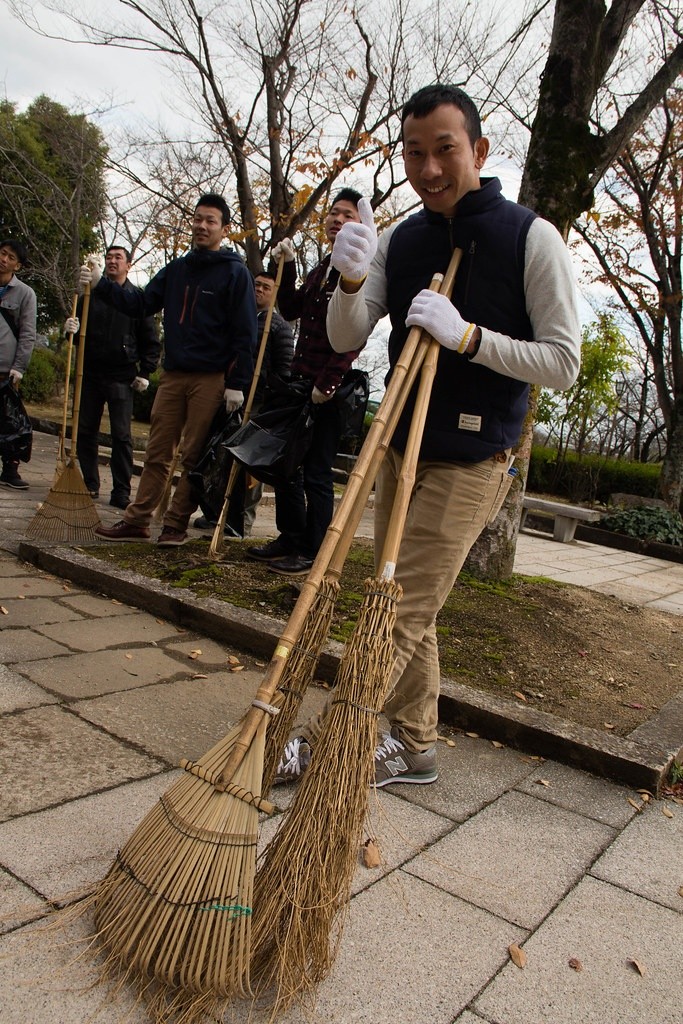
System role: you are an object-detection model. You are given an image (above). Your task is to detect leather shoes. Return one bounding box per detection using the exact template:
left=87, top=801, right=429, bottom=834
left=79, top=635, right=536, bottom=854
left=93, top=519, right=152, bottom=543
left=156, top=524, right=191, bottom=546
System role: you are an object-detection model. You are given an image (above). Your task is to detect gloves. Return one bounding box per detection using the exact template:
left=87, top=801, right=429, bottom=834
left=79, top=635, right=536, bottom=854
left=311, top=386, right=336, bottom=404
left=8, top=368, right=24, bottom=384
left=223, top=388, right=244, bottom=414
left=79, top=257, right=103, bottom=289
left=270, top=237, right=296, bottom=265
left=405, top=289, right=476, bottom=354
left=330, top=196, right=379, bottom=284
left=130, top=376, right=149, bottom=393
left=64, top=317, right=80, bottom=335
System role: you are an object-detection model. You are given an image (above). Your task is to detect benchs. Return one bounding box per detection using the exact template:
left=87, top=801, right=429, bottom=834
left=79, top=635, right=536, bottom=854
left=522, top=496, right=601, bottom=543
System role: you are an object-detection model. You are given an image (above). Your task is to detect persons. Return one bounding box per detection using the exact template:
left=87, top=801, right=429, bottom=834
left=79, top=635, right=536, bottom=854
left=193, top=274, right=295, bottom=536
left=246, top=188, right=366, bottom=576
left=0, top=240, right=37, bottom=489
left=65, top=246, right=161, bottom=510
left=271, top=83, right=581, bottom=787
left=80, top=193, right=256, bottom=548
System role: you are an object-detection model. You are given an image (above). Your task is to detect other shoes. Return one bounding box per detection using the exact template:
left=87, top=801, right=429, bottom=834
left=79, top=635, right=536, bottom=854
left=87, top=490, right=99, bottom=499
left=109, top=495, right=131, bottom=510
left=194, top=516, right=217, bottom=530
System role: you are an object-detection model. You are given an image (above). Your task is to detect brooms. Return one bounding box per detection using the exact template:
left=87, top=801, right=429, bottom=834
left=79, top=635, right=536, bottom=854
left=22, top=246, right=471, bottom=1024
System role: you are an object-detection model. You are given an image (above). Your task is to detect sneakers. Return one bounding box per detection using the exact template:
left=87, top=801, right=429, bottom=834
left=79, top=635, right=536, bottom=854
left=271, top=734, right=310, bottom=787
left=369, top=724, right=440, bottom=788
left=265, top=551, right=316, bottom=576
left=0, top=472, right=30, bottom=489
left=243, top=539, right=290, bottom=561
